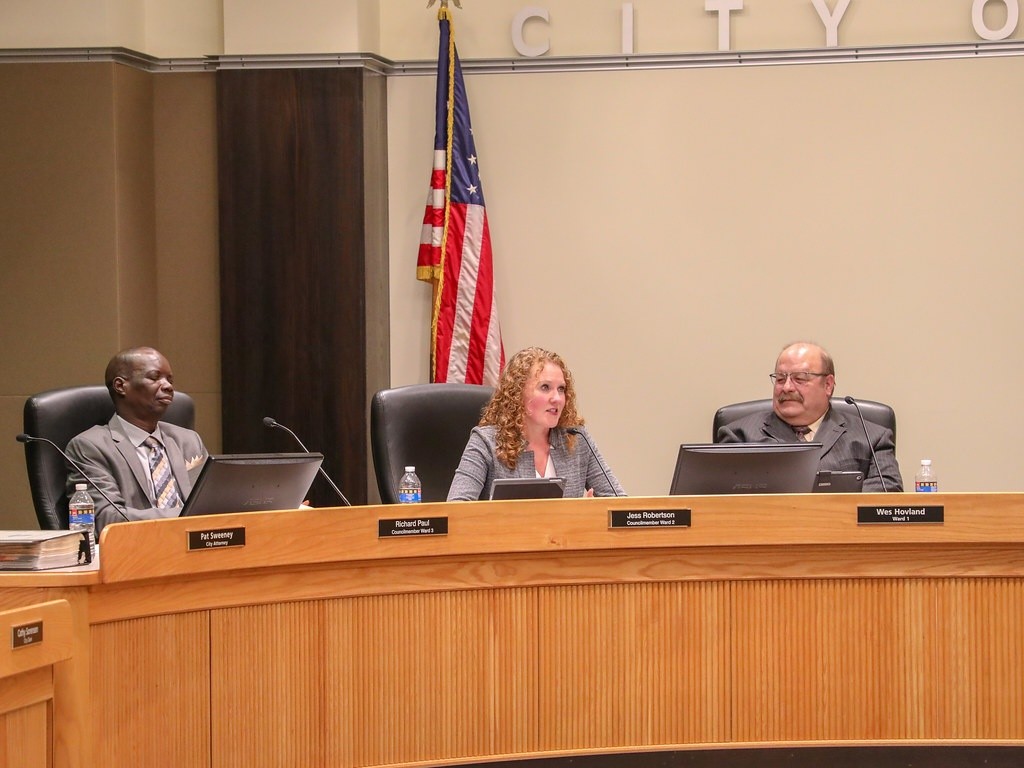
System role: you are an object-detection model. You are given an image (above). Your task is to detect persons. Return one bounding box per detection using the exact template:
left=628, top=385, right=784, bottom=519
left=722, top=342, right=904, bottom=492
left=446, top=346, right=627, bottom=502
left=65, top=347, right=309, bottom=539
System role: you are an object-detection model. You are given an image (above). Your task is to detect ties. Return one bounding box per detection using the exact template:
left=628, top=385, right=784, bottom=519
left=794, top=425, right=810, bottom=442
left=144, top=436, right=181, bottom=510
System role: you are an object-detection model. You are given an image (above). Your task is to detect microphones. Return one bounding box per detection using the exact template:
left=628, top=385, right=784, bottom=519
left=844, top=396, right=888, bottom=494
left=16, top=434, right=132, bottom=522
left=263, top=417, right=352, bottom=507
left=567, top=427, right=620, bottom=497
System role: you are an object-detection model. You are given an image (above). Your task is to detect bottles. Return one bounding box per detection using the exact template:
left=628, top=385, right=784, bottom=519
left=398, top=465, right=422, bottom=504
left=69, top=484, right=95, bottom=564
left=915, top=459, right=938, bottom=492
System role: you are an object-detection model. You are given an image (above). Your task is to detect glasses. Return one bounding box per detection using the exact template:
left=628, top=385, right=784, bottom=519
left=769, top=370, right=831, bottom=387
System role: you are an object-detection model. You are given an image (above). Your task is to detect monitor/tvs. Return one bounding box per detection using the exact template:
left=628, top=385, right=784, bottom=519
left=177, top=452, right=325, bottom=518
left=670, top=443, right=824, bottom=495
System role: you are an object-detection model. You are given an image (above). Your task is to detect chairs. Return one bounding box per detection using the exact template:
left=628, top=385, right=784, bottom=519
left=713, top=399, right=896, bottom=453
left=22, top=383, right=195, bottom=529
left=370, top=383, right=494, bottom=503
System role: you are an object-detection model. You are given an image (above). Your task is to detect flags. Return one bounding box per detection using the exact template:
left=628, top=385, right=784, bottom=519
left=416, top=5, right=506, bottom=388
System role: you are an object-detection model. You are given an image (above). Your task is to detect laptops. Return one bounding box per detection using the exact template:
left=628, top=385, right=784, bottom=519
left=813, top=470, right=864, bottom=493
left=489, top=477, right=567, bottom=499
